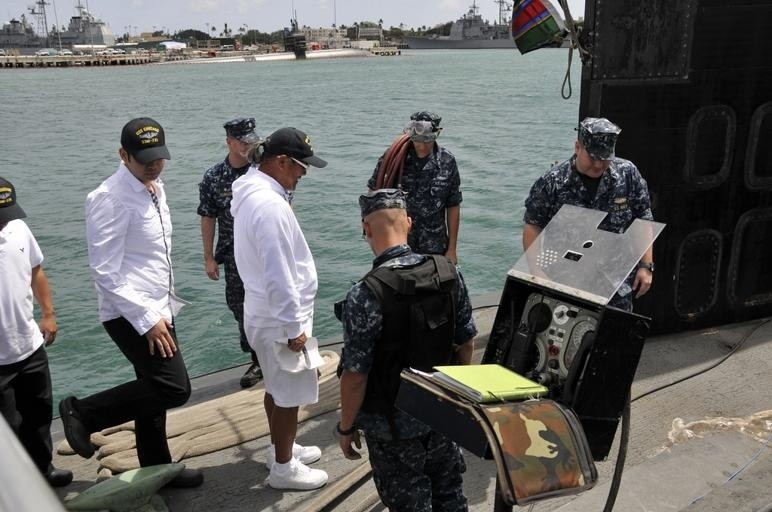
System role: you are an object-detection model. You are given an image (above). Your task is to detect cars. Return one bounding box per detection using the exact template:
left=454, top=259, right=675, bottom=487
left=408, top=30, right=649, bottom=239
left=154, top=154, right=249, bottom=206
left=243, top=45, right=258, bottom=51
left=36, top=50, right=49, bottom=57
left=0, top=49, right=6, bottom=56
left=58, top=49, right=73, bottom=56
left=95, top=48, right=126, bottom=56
left=139, top=48, right=149, bottom=54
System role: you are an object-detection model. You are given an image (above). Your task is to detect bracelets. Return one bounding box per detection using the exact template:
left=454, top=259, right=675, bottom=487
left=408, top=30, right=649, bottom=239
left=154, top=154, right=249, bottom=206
left=336, top=421, right=361, bottom=434
left=640, top=262, right=655, bottom=272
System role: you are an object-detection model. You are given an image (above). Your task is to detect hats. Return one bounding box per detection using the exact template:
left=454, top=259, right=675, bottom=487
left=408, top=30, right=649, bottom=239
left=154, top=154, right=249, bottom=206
left=578, top=116, right=622, bottom=162
left=358, top=187, right=410, bottom=236
left=264, top=125, right=329, bottom=170
left=0, top=176, right=28, bottom=225
left=120, top=116, right=171, bottom=166
left=407, top=109, right=442, bottom=144
left=222, top=117, right=263, bottom=145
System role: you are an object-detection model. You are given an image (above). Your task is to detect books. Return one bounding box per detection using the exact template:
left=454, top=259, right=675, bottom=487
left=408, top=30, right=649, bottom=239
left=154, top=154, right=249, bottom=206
left=428, top=363, right=549, bottom=403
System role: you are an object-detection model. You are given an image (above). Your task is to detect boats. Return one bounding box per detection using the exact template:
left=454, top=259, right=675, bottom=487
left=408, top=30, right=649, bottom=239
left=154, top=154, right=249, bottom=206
left=401, top=1, right=573, bottom=51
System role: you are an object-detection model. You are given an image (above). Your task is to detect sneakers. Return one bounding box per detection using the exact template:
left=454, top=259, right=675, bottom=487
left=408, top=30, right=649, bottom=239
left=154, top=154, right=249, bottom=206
left=42, top=461, right=74, bottom=491
left=239, top=363, right=264, bottom=387
left=267, top=456, right=329, bottom=491
left=265, top=440, right=322, bottom=470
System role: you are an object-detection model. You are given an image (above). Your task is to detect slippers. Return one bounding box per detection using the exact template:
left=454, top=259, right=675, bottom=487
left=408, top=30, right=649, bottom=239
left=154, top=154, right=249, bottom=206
left=158, top=466, right=205, bottom=492
left=58, top=394, right=96, bottom=460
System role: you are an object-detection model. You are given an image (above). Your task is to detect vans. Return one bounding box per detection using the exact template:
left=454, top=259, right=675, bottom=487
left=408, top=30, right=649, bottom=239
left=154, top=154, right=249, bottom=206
left=221, top=45, right=234, bottom=51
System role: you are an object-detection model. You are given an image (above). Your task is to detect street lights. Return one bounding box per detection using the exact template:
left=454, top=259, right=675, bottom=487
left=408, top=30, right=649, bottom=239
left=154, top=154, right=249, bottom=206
left=152, top=25, right=156, bottom=33
left=123, top=24, right=127, bottom=40
left=128, top=24, right=132, bottom=42
left=134, top=25, right=138, bottom=41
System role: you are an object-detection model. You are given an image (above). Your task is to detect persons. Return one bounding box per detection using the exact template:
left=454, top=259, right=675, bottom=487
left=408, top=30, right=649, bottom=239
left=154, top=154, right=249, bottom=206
left=195, top=120, right=262, bottom=390
left=367, top=110, right=464, bottom=271
left=227, top=128, right=329, bottom=490
left=0, top=174, right=75, bottom=488
left=333, top=186, right=477, bottom=512
left=58, top=116, right=204, bottom=488
left=522, top=117, right=656, bottom=313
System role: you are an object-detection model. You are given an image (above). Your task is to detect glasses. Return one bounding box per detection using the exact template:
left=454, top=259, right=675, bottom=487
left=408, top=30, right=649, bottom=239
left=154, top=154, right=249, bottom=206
left=404, top=120, right=434, bottom=137
